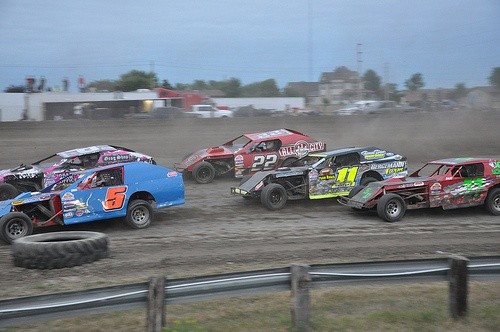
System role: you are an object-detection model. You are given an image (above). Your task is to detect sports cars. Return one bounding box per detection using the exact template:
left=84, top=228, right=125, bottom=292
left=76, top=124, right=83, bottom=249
left=0, top=161, right=186, bottom=248
left=336, top=155, right=500, bottom=222
left=173, top=127, right=328, bottom=186
left=0, top=144, right=157, bottom=204
left=230, top=144, right=408, bottom=212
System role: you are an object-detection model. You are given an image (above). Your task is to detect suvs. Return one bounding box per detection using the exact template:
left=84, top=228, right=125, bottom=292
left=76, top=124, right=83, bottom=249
left=188, top=104, right=233, bottom=118
left=356, top=100, right=397, bottom=114
left=152, top=106, right=203, bottom=119
